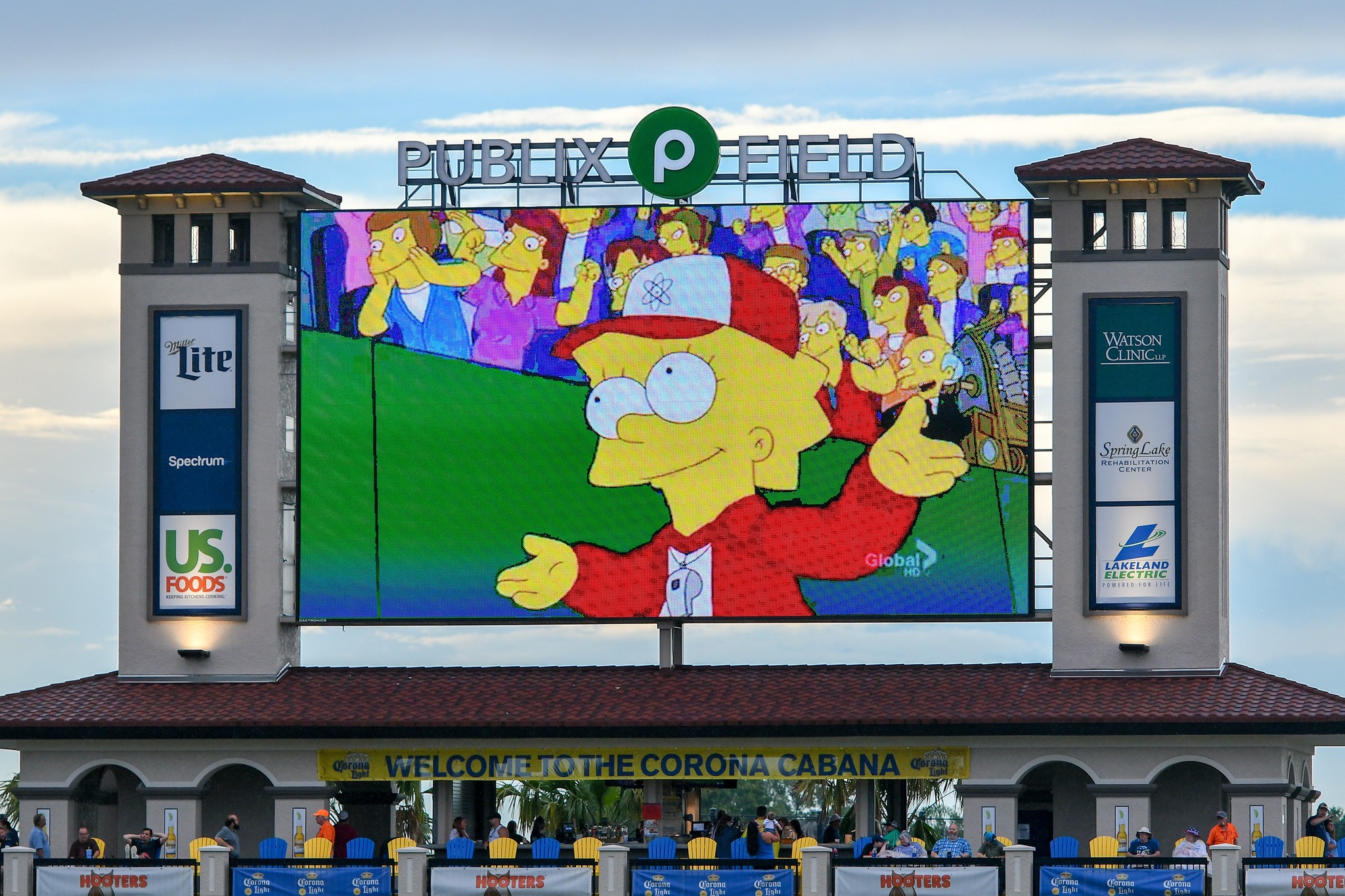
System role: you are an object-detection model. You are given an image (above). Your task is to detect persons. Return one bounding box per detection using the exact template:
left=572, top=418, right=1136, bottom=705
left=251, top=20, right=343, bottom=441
left=449, top=816, right=477, bottom=845
left=67, top=827, right=101, bottom=868
left=1167, top=827, right=1212, bottom=878
left=123, top=828, right=168, bottom=859
left=702, top=809, right=807, bottom=870
left=507, top=821, right=530, bottom=845
left=530, top=816, right=546, bottom=844
left=858, top=835, right=892, bottom=867
left=875, top=819, right=883, bottom=835
left=883, top=820, right=901, bottom=852
left=29, top=814, right=51, bottom=869
left=1324, top=820, right=1339, bottom=868
left=977, top=832, right=1006, bottom=895
left=823, top=814, right=844, bottom=844
left=891, top=830, right=928, bottom=868
left=333, top=811, right=359, bottom=868
left=0, top=814, right=19, bottom=874
left=931, top=823, right=973, bottom=867
left=314, top=809, right=335, bottom=859
left=1305, top=803, right=1334, bottom=858
left=214, top=814, right=241, bottom=896
left=1206, top=811, right=1239, bottom=861
left=1126, top=827, right=1161, bottom=870
left=482, top=812, right=510, bottom=850
left=638, top=821, right=644, bottom=843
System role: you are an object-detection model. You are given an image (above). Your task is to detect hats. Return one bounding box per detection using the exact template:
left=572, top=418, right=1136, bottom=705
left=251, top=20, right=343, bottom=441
left=1184, top=827, right=1199, bottom=836
left=313, top=810, right=329, bottom=818
left=1136, top=827, right=1153, bottom=840
left=984, top=832, right=998, bottom=845
left=487, top=812, right=501, bottom=819
left=883, top=820, right=897, bottom=828
left=872, top=834, right=887, bottom=843
left=1319, top=802, right=1329, bottom=811
left=1216, top=811, right=1227, bottom=818
left=829, top=814, right=844, bottom=822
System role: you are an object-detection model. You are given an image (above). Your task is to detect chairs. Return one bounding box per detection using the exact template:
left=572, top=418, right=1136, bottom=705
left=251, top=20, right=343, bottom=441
left=296, top=837, right=333, bottom=868
left=1050, top=835, right=1345, bottom=869
left=340, top=838, right=375, bottom=868
left=481, top=838, right=519, bottom=868
left=437, top=837, right=476, bottom=867
left=251, top=838, right=288, bottom=868
left=180, top=837, right=218, bottom=896
left=649, top=836, right=817, bottom=896
left=126, top=839, right=162, bottom=867
left=523, top=837, right=561, bottom=868
left=853, top=834, right=1013, bottom=858
left=382, top=838, right=417, bottom=896
left=91, top=838, right=106, bottom=868
left=567, top=837, right=602, bottom=896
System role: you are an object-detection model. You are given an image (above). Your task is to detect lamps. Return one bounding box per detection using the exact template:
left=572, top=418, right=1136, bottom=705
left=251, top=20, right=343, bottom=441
left=1119, top=644, right=1149, bottom=654
left=177, top=649, right=210, bottom=659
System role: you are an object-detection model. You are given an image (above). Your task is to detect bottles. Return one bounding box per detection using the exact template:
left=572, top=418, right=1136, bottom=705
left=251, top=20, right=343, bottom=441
left=912, top=847, right=917, bottom=858
left=1251, top=814, right=1262, bottom=856
left=583, top=821, right=628, bottom=844
left=1116, top=815, right=1127, bottom=857
left=166, top=820, right=176, bottom=858
left=294, top=820, right=304, bottom=857
left=986, top=815, right=992, bottom=832
left=872, top=846, right=877, bottom=858
left=833, top=848, right=838, bottom=858
left=947, top=846, right=952, bottom=858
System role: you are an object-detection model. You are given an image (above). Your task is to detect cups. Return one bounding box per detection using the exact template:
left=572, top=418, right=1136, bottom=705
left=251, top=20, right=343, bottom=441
left=835, top=839, right=840, bottom=844
left=478, top=840, right=483, bottom=848
left=86, top=849, right=92, bottom=858
left=942, top=851, right=947, bottom=858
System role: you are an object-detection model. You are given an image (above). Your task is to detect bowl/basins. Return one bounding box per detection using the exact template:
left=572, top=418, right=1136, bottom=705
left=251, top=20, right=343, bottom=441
left=671, top=833, right=692, bottom=844
